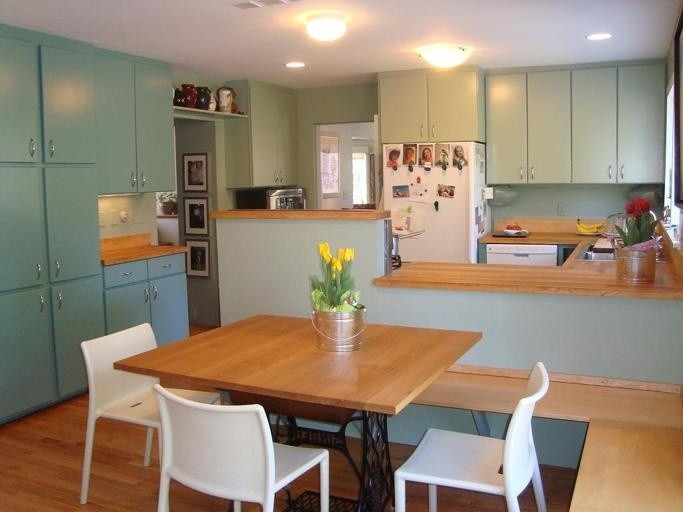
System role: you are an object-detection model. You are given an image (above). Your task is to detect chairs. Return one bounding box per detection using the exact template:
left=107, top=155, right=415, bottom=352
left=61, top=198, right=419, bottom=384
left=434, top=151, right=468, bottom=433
left=80, top=320, right=222, bottom=510
left=394, top=360, right=550, bottom=511
left=152, top=380, right=331, bottom=511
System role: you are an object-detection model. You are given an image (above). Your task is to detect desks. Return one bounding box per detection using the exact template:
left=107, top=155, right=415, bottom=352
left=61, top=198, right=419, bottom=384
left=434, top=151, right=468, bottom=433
left=113, top=314, right=483, bottom=512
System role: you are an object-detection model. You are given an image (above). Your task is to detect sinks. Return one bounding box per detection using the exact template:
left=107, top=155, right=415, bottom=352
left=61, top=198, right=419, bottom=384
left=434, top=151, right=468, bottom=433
left=577, top=241, right=616, bottom=263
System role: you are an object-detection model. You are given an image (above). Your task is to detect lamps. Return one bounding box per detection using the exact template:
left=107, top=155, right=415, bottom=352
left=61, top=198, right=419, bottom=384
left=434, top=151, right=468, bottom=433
left=305, top=13, right=347, bottom=43
left=419, top=44, right=472, bottom=70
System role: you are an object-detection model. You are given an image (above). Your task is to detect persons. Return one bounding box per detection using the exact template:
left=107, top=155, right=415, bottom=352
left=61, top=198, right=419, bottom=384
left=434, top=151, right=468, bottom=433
left=435, top=149, right=449, bottom=165
left=403, top=148, right=414, bottom=165
left=420, top=148, right=433, bottom=165
left=436, top=186, right=454, bottom=197
left=453, top=145, right=467, bottom=168
left=387, top=150, right=400, bottom=167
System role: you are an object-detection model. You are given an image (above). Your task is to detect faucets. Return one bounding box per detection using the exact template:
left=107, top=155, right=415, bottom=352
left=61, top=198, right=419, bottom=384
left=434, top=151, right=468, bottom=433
left=605, top=209, right=660, bottom=226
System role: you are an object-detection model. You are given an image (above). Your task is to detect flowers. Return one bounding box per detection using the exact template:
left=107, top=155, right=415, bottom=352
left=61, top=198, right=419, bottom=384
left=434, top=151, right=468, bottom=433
left=614, top=199, right=657, bottom=247
left=308, top=241, right=365, bottom=311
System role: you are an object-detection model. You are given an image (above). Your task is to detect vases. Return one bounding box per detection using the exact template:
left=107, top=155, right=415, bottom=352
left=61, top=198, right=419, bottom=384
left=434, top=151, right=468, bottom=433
left=614, top=248, right=657, bottom=287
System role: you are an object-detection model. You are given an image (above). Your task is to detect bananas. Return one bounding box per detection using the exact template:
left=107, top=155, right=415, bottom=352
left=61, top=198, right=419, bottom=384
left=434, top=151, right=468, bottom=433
left=576, top=218, right=605, bottom=232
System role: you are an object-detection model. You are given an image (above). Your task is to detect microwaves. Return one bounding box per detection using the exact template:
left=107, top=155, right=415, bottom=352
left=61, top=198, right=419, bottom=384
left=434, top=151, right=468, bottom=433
left=236, top=186, right=307, bottom=210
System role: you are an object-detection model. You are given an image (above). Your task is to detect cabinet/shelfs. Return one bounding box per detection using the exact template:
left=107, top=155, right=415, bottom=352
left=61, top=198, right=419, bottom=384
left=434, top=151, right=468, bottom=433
left=1, top=276, right=107, bottom=424
left=100, top=48, right=177, bottom=195
left=378, top=67, right=486, bottom=144
left=0, top=166, right=102, bottom=293
left=223, top=80, right=298, bottom=189
left=104, top=250, right=190, bottom=347
left=486, top=244, right=558, bottom=266
left=485, top=57, right=666, bottom=185
left=0, top=22, right=106, bottom=165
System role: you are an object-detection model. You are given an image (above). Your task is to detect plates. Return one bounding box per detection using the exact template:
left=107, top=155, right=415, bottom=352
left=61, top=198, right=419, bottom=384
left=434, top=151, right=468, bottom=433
left=576, top=233, right=602, bottom=236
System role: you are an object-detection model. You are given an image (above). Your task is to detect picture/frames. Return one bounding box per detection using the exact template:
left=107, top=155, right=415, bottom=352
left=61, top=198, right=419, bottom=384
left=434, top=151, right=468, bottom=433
left=184, top=239, right=211, bottom=279
left=182, top=196, right=209, bottom=237
left=182, top=152, right=209, bottom=192
